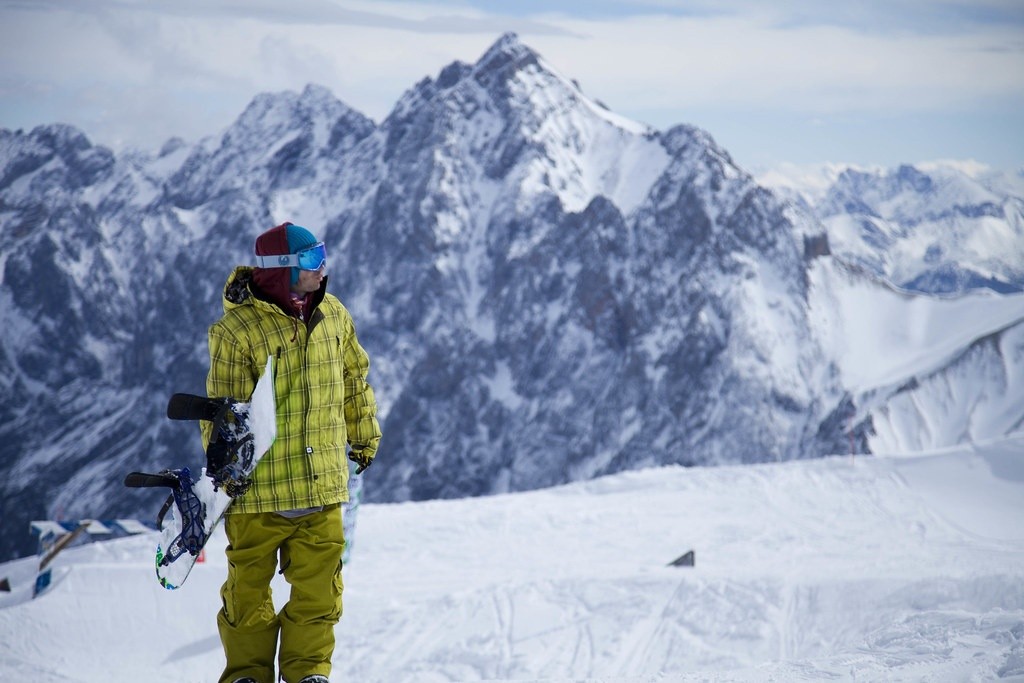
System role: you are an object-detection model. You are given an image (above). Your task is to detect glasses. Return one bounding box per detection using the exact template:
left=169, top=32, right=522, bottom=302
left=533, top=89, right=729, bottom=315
left=296, top=241, right=328, bottom=271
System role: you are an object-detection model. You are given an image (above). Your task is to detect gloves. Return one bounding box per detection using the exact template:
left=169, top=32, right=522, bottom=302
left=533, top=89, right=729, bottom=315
left=349, top=450, right=372, bottom=474
left=211, top=465, right=253, bottom=499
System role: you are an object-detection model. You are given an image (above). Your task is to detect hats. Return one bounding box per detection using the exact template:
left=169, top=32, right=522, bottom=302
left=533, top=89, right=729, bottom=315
left=286, top=224, right=317, bottom=284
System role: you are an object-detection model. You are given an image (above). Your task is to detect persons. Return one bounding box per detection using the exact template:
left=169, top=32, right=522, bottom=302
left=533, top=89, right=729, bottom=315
left=199, top=223, right=382, bottom=683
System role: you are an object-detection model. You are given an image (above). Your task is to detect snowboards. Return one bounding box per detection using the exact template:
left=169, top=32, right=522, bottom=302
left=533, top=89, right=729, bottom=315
left=153, top=354, right=279, bottom=590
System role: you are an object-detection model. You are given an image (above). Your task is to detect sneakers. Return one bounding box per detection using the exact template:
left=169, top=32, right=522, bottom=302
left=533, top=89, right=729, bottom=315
left=300, top=675, right=329, bottom=683
left=231, top=676, right=258, bottom=683
